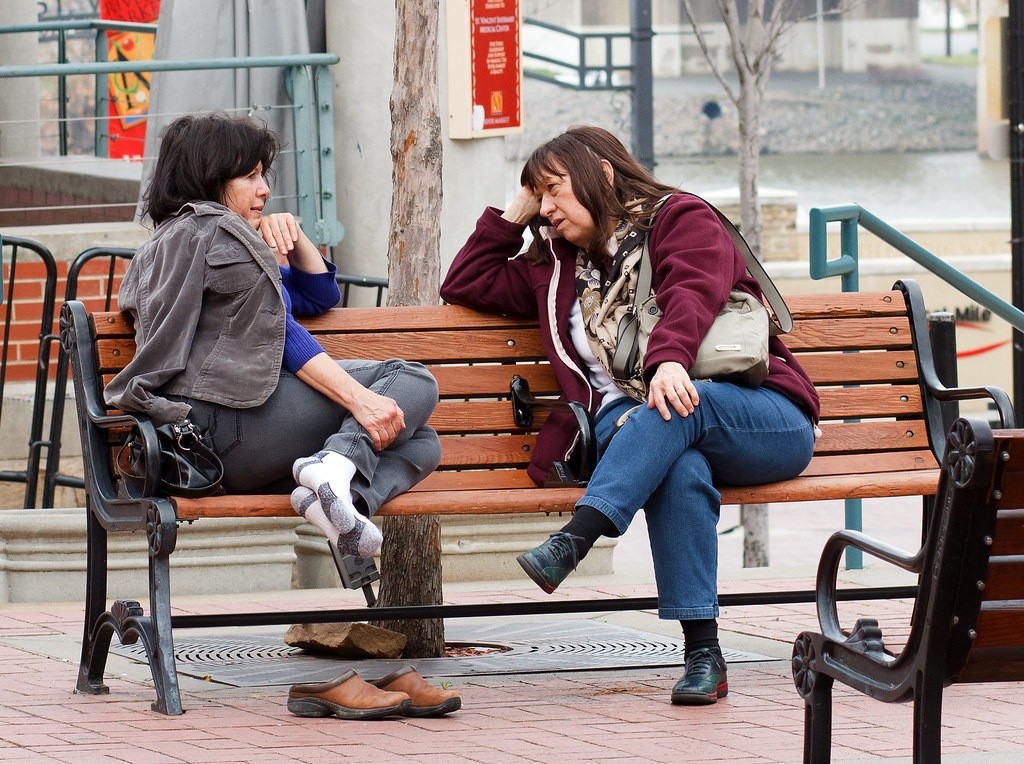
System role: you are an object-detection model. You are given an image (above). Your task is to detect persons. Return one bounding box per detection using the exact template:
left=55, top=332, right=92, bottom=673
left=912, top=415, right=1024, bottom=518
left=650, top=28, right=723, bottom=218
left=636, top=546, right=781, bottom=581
left=441, top=126, right=820, bottom=705
left=103, top=115, right=441, bottom=559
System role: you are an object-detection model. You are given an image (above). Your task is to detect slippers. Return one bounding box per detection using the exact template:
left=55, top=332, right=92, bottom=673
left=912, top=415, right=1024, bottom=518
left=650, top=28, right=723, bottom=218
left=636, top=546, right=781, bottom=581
left=287, top=667, right=461, bottom=720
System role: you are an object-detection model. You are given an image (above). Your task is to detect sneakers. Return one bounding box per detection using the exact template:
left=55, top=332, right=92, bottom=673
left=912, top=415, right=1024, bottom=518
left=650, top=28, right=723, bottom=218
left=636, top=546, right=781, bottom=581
left=517, top=532, right=585, bottom=595
left=672, top=646, right=729, bottom=705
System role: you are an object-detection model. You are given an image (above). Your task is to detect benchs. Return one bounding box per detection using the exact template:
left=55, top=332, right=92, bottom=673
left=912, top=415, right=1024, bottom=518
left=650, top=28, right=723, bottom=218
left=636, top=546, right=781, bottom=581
left=57, top=277, right=1017, bottom=716
left=789, top=418, right=1024, bottom=764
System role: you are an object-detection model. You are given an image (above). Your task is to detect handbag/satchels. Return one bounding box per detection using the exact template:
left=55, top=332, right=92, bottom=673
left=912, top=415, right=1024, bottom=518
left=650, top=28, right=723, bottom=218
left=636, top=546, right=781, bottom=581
left=612, top=291, right=771, bottom=382
left=116, top=418, right=223, bottom=497
